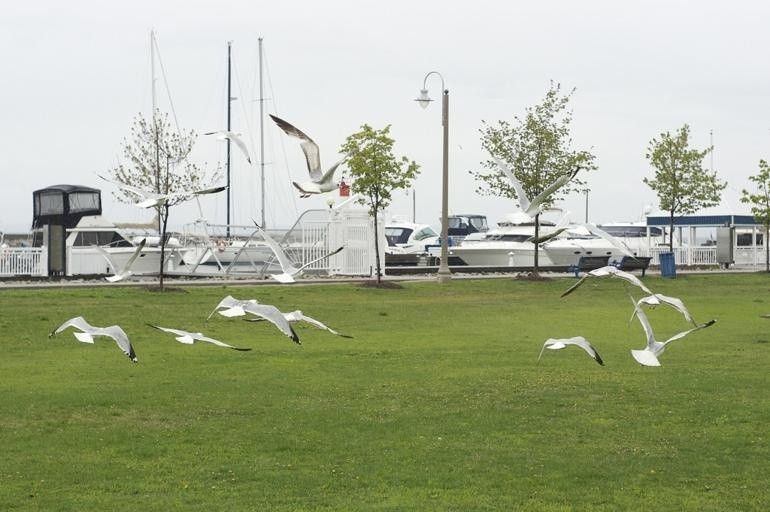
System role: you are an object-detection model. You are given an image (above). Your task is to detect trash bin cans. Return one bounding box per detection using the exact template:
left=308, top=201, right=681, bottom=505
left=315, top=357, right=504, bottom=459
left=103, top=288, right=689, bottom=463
left=659, top=253, right=676, bottom=277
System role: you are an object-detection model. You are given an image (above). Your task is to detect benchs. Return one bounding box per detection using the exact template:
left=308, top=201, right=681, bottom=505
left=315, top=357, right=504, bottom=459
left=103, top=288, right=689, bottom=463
left=566, top=255, right=654, bottom=279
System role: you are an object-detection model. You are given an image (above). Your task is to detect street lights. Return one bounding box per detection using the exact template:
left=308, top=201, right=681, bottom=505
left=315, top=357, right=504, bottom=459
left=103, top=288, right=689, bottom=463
left=583, top=188, right=592, bottom=224
left=413, top=69, right=451, bottom=281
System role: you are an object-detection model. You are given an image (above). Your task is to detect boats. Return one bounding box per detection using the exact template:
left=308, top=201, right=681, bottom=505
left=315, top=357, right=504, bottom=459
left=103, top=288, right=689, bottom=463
left=32, top=185, right=676, bottom=279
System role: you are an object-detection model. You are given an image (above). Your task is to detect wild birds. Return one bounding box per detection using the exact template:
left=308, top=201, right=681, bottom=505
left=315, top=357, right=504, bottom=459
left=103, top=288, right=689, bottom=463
left=144, top=320, right=254, bottom=357
left=270, top=114, right=360, bottom=198
left=94, top=172, right=228, bottom=208
left=47, top=313, right=141, bottom=365
left=524, top=215, right=716, bottom=368
left=204, top=130, right=252, bottom=166
left=205, top=294, right=354, bottom=348
left=490, top=155, right=581, bottom=219
